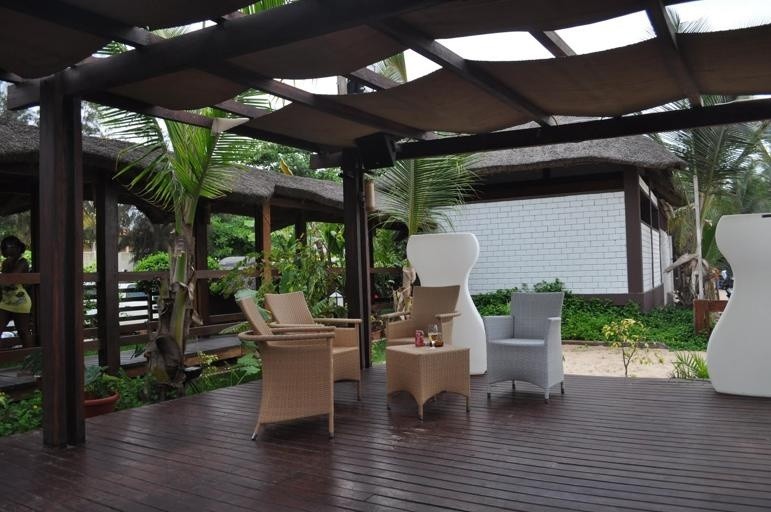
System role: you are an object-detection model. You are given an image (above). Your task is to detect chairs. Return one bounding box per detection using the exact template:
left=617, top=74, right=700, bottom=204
left=386, top=284, right=461, bottom=400
left=264, top=292, right=362, bottom=399
left=235, top=296, right=336, bottom=439
left=483, top=291, right=565, bottom=405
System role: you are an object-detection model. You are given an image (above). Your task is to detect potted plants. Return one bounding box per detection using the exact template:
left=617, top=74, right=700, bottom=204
left=84, top=365, right=121, bottom=418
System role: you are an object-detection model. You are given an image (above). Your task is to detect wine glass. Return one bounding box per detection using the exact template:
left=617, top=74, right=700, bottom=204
left=427, top=324, right=438, bottom=350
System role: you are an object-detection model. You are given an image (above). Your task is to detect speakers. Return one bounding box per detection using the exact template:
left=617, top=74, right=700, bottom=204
left=356, top=132, right=397, bottom=168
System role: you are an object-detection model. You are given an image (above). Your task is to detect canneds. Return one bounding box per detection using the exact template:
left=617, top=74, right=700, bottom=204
left=415, top=330, right=424, bottom=346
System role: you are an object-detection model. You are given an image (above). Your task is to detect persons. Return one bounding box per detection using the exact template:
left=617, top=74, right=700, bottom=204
left=1, top=234, right=34, bottom=351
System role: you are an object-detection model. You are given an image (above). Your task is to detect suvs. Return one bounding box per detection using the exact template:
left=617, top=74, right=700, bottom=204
left=82, top=279, right=161, bottom=328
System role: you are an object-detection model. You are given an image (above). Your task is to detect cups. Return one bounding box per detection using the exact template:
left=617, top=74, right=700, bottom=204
left=430, top=334, right=444, bottom=347
